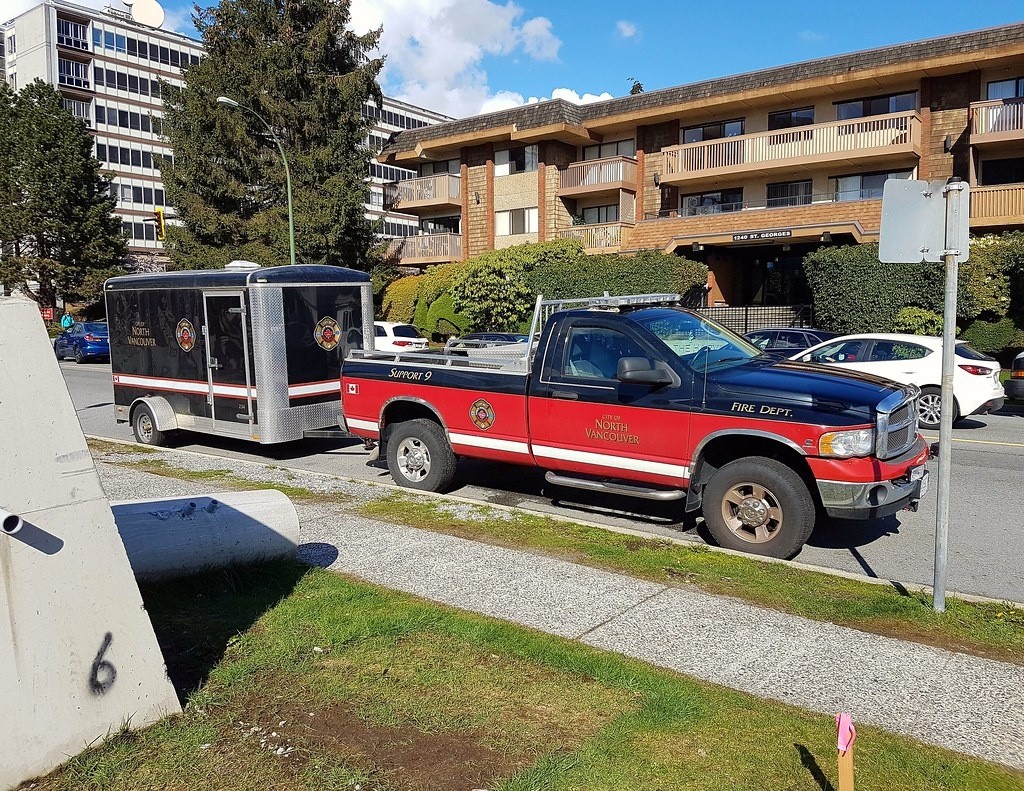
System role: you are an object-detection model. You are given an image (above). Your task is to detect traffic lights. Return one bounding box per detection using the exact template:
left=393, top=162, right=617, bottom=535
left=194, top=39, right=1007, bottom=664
left=154, top=207, right=165, bottom=241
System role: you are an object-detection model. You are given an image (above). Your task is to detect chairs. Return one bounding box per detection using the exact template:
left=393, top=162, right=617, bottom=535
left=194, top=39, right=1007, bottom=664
left=569, top=336, right=605, bottom=378
left=876, top=342, right=893, bottom=360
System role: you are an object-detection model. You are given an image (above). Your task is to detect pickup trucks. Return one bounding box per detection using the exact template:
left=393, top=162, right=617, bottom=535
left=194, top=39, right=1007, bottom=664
left=338, top=288, right=940, bottom=561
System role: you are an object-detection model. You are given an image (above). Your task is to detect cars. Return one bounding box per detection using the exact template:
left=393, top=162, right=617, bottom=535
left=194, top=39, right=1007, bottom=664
left=54, top=320, right=110, bottom=365
left=1004, top=351, right=1024, bottom=401
left=775, top=332, right=1005, bottom=430
left=445, top=330, right=540, bottom=356
left=717, top=327, right=863, bottom=363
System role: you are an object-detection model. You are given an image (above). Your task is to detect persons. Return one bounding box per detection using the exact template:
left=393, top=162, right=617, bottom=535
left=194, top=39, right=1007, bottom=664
left=61, top=311, right=75, bottom=331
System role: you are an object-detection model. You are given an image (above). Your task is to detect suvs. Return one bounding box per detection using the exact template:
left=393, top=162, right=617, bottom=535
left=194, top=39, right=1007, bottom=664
left=373, top=321, right=430, bottom=354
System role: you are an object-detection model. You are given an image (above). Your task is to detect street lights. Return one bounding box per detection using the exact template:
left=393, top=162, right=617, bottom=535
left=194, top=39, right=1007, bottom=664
left=217, top=97, right=296, bottom=266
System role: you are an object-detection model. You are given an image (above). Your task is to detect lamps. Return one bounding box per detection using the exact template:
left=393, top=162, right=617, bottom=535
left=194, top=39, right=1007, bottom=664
left=475, top=192, right=479, bottom=202
left=654, top=173, right=659, bottom=184
left=783, top=244, right=790, bottom=251
left=945, top=134, right=951, bottom=149
